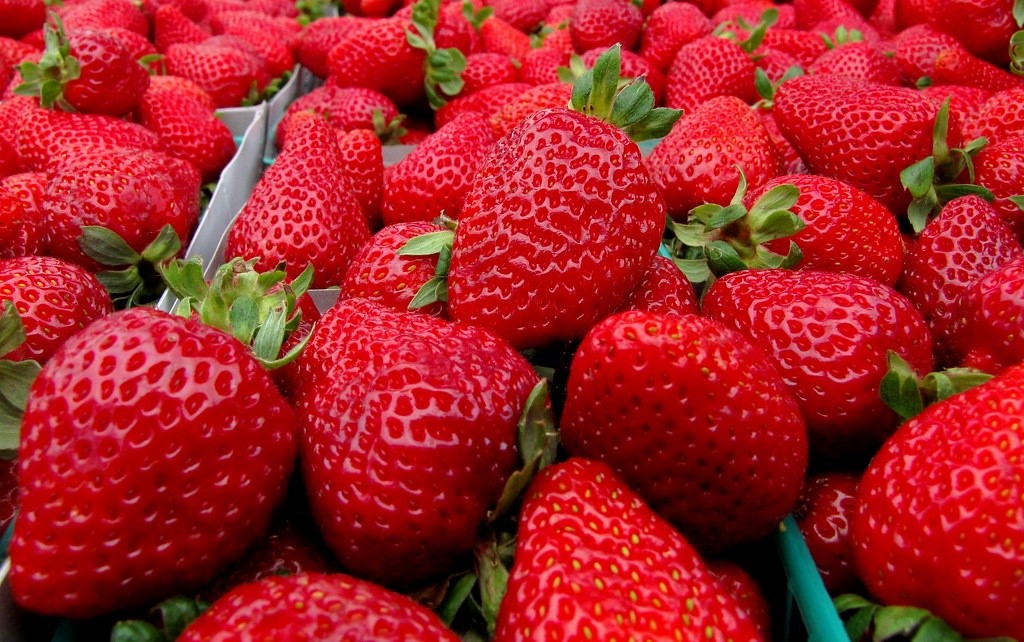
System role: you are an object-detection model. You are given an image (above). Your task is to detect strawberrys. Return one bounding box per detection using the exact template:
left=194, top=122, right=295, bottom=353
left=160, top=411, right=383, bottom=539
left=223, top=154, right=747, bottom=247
left=0, top=0, right=1024, bottom=642
